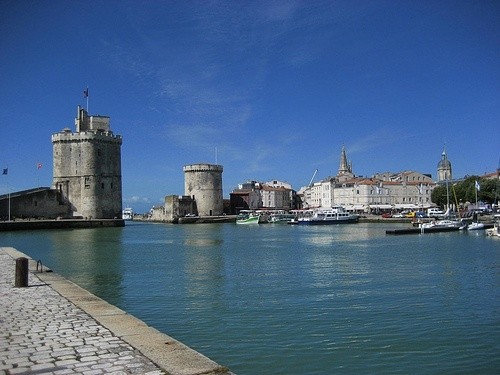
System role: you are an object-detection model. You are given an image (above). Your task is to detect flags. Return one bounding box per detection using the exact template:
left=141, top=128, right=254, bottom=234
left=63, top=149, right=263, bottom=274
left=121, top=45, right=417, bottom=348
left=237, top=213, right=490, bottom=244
left=2, top=169, right=8, bottom=176
left=83, top=88, right=87, bottom=98
left=38, top=164, right=42, bottom=169
left=475, top=180, right=480, bottom=192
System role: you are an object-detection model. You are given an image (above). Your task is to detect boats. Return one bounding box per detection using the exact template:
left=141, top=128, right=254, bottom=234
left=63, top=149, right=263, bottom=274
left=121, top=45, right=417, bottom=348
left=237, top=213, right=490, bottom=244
left=268, top=213, right=295, bottom=223
left=122, top=208, right=134, bottom=221
left=419, top=220, right=463, bottom=228
left=236, top=212, right=268, bottom=225
left=459, top=222, right=484, bottom=230
left=298, top=212, right=360, bottom=225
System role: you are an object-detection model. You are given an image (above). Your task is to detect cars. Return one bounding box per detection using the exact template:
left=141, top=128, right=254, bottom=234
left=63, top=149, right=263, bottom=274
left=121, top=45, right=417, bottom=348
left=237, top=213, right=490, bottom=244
left=382, top=207, right=484, bottom=219
